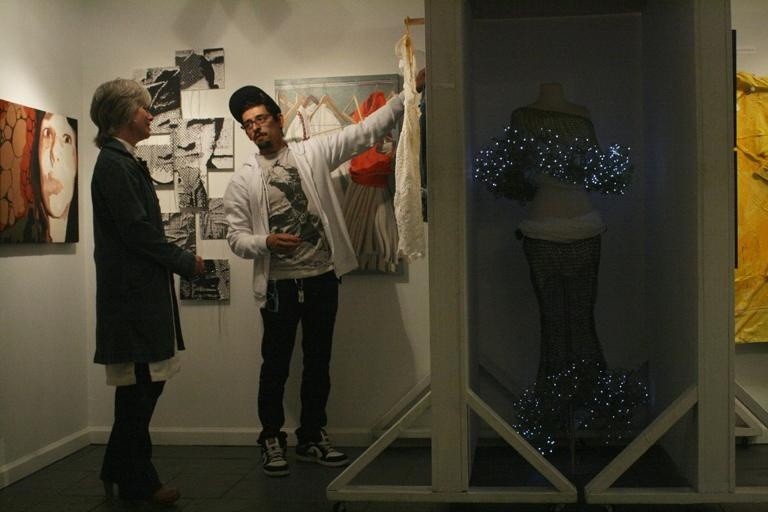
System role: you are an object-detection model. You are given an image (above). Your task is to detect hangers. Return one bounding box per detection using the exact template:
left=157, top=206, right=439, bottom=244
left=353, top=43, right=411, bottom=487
left=403, top=14, right=415, bottom=63
left=273, top=79, right=400, bottom=133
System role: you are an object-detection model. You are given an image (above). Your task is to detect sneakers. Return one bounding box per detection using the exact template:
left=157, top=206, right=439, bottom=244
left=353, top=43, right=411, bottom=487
left=260, top=437, right=290, bottom=477
left=295, top=429, right=349, bottom=467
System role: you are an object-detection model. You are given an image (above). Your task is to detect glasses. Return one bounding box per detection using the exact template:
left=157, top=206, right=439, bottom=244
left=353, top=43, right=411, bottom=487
left=240, top=113, right=272, bottom=131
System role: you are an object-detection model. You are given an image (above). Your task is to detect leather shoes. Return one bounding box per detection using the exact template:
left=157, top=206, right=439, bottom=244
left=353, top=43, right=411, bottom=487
left=149, top=485, right=180, bottom=504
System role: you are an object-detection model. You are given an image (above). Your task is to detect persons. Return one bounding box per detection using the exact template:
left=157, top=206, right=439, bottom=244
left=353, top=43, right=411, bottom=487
left=224, top=68, right=425, bottom=477
left=135, top=108, right=224, bottom=185
left=88, top=78, right=205, bottom=505
left=472, top=83, right=652, bottom=459
left=0, top=110, right=78, bottom=243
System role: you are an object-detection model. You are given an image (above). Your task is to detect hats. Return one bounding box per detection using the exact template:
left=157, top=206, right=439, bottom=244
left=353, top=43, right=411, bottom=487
left=229, top=85, right=280, bottom=125
